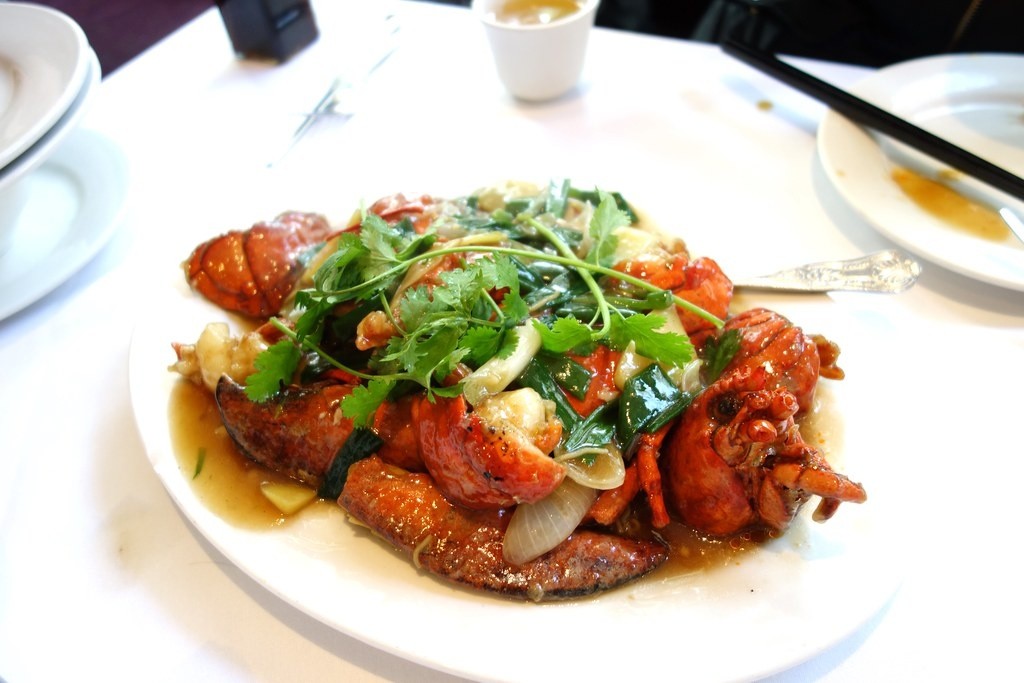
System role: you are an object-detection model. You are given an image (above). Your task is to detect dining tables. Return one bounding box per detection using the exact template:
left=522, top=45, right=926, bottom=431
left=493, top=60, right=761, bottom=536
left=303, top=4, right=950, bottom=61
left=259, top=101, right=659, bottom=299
left=0, top=0, right=1024, bottom=683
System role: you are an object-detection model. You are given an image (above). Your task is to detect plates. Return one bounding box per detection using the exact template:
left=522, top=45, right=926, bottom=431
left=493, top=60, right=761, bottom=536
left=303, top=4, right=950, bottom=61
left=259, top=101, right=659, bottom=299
left=130, top=299, right=900, bottom=682
left=816, top=53, right=1024, bottom=295
left=0, top=45, right=101, bottom=191
left=0, top=131, right=129, bottom=318
left=1, top=2, right=90, bottom=170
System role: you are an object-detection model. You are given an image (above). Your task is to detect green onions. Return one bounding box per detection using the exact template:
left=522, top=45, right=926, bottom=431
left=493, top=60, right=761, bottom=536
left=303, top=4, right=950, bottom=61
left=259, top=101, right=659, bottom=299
left=322, top=189, right=698, bottom=505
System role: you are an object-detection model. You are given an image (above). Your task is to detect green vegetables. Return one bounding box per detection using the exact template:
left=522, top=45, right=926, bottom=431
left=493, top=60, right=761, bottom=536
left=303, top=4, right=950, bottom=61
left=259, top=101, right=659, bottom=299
left=244, top=188, right=730, bottom=432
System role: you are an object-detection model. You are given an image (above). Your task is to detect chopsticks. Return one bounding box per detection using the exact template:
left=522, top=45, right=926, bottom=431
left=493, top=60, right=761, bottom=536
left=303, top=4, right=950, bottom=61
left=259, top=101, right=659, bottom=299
left=721, top=34, right=1024, bottom=203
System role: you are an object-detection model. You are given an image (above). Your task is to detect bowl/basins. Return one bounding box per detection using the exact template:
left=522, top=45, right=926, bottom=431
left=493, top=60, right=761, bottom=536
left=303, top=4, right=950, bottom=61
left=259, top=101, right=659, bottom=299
left=472, top=0, right=600, bottom=103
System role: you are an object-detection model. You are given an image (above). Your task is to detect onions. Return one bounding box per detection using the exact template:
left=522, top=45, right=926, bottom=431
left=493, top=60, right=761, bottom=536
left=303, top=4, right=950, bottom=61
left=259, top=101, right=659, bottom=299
left=502, top=436, right=625, bottom=564
